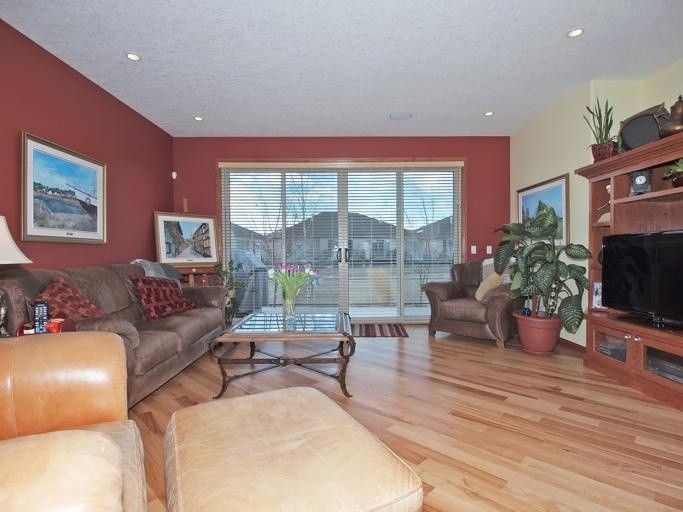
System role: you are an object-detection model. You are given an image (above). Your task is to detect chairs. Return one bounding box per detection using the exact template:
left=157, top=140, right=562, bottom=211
left=420, top=260, right=526, bottom=349
left=0, top=331, right=149, bottom=512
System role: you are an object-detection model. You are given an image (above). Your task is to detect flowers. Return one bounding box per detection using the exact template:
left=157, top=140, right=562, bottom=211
left=271, top=262, right=320, bottom=297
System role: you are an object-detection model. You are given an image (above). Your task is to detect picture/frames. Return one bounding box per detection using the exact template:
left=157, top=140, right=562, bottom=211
left=20, top=130, right=107, bottom=245
left=589, top=280, right=609, bottom=312
left=515, top=173, right=570, bottom=251
left=154, top=211, right=218, bottom=266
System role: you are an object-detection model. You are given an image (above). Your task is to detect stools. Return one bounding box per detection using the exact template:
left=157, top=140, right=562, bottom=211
left=164, top=387, right=424, bottom=512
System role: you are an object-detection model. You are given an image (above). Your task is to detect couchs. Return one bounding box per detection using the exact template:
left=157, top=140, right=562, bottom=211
left=0, top=264, right=226, bottom=411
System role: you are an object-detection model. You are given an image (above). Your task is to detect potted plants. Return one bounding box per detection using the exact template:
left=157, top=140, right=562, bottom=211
left=494, top=206, right=593, bottom=356
left=663, top=158, right=683, bottom=187
left=583, top=96, right=621, bottom=161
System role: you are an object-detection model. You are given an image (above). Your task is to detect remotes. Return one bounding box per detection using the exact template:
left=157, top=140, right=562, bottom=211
left=33, top=301, right=50, bottom=334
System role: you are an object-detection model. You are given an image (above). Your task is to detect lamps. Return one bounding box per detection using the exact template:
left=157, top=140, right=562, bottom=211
left=0, top=216, right=34, bottom=337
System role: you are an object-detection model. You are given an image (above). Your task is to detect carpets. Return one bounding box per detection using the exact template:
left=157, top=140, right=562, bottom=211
left=350, top=323, right=409, bottom=337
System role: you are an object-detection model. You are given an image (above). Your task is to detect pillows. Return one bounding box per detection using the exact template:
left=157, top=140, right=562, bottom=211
left=474, top=272, right=504, bottom=302
left=30, top=276, right=107, bottom=320
left=136, top=277, right=197, bottom=320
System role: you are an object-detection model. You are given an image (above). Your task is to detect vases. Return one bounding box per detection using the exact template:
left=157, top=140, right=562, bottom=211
left=284, top=295, right=295, bottom=316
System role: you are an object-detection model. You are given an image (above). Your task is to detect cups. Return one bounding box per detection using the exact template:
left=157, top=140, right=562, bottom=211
left=43, top=318, right=64, bottom=333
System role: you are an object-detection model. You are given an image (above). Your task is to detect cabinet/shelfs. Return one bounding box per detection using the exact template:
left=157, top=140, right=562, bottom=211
left=573, top=132, right=682, bottom=413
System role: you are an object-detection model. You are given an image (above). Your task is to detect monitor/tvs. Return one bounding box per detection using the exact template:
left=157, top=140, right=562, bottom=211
left=601, top=229, right=682, bottom=330
